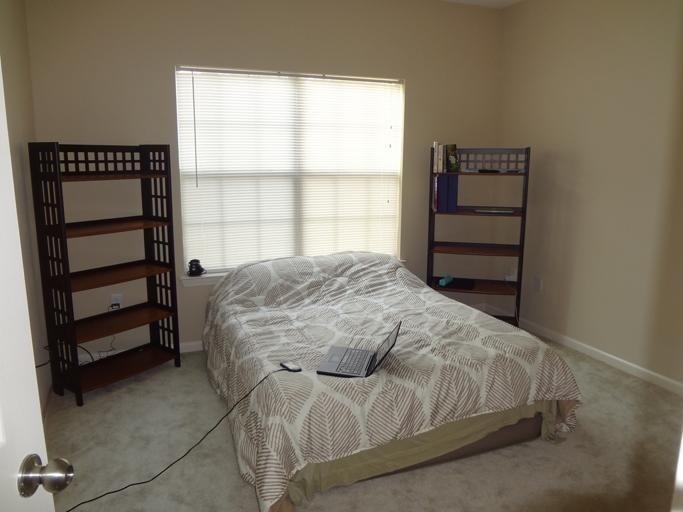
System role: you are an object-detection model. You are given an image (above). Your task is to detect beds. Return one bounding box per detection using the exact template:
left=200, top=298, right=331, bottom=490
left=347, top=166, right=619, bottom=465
left=202, top=251, right=582, bottom=511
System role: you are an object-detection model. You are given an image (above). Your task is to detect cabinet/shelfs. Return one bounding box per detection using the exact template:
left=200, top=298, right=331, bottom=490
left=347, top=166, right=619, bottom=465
left=27, top=142, right=180, bottom=408
left=427, top=147, right=532, bottom=327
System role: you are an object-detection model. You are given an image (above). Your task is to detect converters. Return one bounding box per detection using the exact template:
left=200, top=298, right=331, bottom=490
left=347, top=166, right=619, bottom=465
left=111, top=302, right=120, bottom=309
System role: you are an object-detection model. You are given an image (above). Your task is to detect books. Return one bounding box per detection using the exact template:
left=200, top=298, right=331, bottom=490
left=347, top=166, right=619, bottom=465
left=433, top=142, right=438, bottom=173
left=443, top=144, right=447, bottom=174
left=447, top=174, right=459, bottom=212
left=438, top=145, right=443, bottom=174
left=434, top=176, right=448, bottom=213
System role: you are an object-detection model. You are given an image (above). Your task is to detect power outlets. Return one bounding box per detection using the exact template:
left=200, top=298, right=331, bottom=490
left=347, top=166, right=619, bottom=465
left=110, top=293, right=122, bottom=309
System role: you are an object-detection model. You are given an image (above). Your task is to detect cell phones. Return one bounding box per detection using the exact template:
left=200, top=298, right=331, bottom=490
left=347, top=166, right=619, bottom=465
left=280, top=360, right=302, bottom=372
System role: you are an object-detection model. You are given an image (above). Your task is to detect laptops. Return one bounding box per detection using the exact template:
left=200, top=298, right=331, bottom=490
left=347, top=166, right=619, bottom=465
left=317, top=321, right=403, bottom=379
